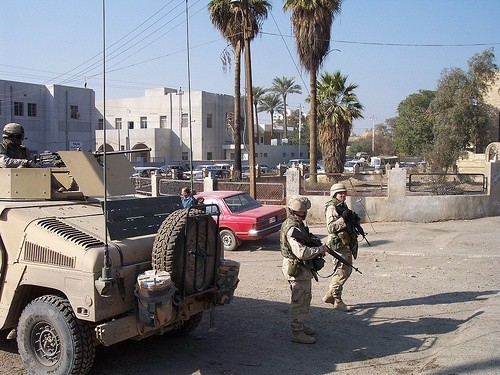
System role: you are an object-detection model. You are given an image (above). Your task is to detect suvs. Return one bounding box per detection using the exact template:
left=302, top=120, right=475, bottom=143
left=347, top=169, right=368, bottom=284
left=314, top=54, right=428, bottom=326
left=0, top=147, right=241, bottom=375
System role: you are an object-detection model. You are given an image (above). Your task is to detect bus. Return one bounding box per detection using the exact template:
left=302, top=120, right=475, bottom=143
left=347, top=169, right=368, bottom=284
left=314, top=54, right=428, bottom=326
left=371, top=155, right=400, bottom=167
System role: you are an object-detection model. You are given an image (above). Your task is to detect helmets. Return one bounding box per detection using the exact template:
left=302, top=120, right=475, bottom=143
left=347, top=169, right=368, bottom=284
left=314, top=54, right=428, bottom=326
left=330, top=184, right=347, bottom=199
left=2, top=123, right=24, bottom=138
left=287, top=196, right=311, bottom=211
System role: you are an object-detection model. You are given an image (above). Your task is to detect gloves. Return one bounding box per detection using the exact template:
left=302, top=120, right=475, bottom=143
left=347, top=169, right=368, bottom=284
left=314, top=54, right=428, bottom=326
left=342, top=210, right=350, bottom=220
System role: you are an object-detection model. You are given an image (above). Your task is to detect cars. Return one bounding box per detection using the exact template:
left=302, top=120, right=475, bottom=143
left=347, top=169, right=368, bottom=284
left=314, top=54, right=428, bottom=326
left=182, top=163, right=232, bottom=180
left=158, top=163, right=185, bottom=174
left=192, top=190, right=287, bottom=251
left=285, top=158, right=321, bottom=171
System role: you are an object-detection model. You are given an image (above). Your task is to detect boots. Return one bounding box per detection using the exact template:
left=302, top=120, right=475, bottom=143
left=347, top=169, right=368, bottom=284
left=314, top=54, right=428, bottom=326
left=334, top=299, right=352, bottom=312
left=322, top=291, right=334, bottom=304
left=302, top=324, right=316, bottom=335
left=289, top=330, right=316, bottom=343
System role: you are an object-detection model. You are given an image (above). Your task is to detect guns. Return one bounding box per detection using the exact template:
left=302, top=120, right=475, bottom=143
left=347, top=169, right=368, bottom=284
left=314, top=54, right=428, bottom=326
left=336, top=201, right=372, bottom=249
left=29, top=146, right=151, bottom=170
left=290, top=227, right=363, bottom=282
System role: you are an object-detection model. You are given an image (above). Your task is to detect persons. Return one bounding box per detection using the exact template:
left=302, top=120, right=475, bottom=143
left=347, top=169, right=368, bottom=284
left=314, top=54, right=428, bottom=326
left=181, top=187, right=196, bottom=209
left=0, top=123, right=43, bottom=169
left=280, top=197, right=328, bottom=343
left=325, top=184, right=358, bottom=311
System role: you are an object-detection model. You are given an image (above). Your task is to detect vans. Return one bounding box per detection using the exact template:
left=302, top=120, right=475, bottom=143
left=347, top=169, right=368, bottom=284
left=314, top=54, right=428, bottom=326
left=344, top=160, right=364, bottom=171
left=134, top=161, right=160, bottom=175
